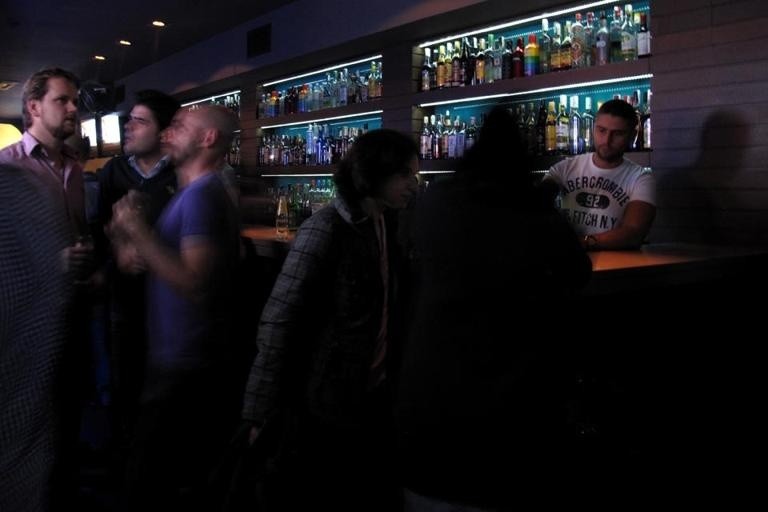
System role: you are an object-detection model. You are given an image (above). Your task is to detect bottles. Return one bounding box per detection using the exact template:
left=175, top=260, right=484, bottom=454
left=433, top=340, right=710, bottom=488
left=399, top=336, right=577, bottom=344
left=264, top=179, right=335, bottom=236
left=551, top=22, right=563, bottom=71
left=493, top=35, right=504, bottom=81
left=421, top=48, right=430, bottom=90
left=538, top=18, right=551, bottom=72
left=554, top=94, right=572, bottom=153
left=476, top=38, right=484, bottom=86
left=259, top=124, right=369, bottom=166
left=612, top=91, right=640, bottom=152
left=468, top=36, right=477, bottom=86
left=502, top=40, right=513, bottom=78
left=609, top=6, right=624, bottom=63
left=582, top=98, right=595, bottom=153
left=620, top=4, right=637, bottom=60
left=190, top=95, right=240, bottom=117
left=437, top=45, right=449, bottom=86
left=459, top=36, right=470, bottom=86
left=420, top=102, right=541, bottom=160
left=561, top=21, right=573, bottom=68
left=572, top=13, right=584, bottom=66
left=543, top=99, right=558, bottom=155
left=484, top=33, right=495, bottom=85
left=257, top=60, right=383, bottom=118
left=636, top=12, right=651, bottom=58
left=524, top=32, right=538, bottom=75
left=584, top=12, right=595, bottom=65
left=432, top=49, right=439, bottom=87
left=642, top=90, right=653, bottom=153
left=596, top=9, right=609, bottom=64
left=451, top=40, right=462, bottom=86
left=443, top=42, right=454, bottom=86
left=569, top=96, right=583, bottom=156
left=513, top=38, right=524, bottom=76
left=225, top=133, right=242, bottom=171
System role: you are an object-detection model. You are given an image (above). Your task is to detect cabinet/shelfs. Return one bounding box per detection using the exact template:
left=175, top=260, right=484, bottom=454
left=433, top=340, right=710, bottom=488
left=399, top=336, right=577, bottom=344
left=179, top=92, right=242, bottom=174
left=413, top=0, right=652, bottom=207
left=258, top=56, right=383, bottom=220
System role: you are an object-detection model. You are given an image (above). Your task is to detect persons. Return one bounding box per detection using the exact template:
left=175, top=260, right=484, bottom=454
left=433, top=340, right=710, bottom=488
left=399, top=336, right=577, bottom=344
left=1, top=66, right=654, bottom=512
